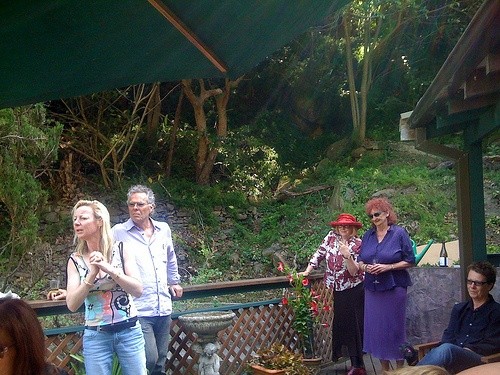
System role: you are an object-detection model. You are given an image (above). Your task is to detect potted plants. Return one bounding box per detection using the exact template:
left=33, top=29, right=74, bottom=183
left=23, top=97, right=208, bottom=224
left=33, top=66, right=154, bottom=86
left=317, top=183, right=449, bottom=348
left=249, top=341, right=313, bottom=375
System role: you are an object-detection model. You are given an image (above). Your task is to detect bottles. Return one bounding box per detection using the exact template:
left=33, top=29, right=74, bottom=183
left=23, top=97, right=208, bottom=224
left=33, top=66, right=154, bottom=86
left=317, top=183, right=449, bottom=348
left=440, top=241, right=448, bottom=267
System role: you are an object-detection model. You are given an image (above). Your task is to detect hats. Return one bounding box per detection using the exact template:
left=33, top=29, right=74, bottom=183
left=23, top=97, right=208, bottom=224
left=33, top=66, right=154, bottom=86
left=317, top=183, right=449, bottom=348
left=330, top=213, right=362, bottom=230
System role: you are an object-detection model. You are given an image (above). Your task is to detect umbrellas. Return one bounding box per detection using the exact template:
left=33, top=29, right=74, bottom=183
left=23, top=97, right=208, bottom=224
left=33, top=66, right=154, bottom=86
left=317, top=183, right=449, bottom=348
left=0, top=0, right=355, bottom=111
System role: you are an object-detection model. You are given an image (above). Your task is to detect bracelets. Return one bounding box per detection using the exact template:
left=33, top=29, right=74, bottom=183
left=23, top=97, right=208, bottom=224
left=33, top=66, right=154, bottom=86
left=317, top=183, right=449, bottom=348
left=82, top=278, right=96, bottom=289
left=113, top=270, right=122, bottom=281
left=344, top=255, right=352, bottom=260
left=391, top=264, right=395, bottom=270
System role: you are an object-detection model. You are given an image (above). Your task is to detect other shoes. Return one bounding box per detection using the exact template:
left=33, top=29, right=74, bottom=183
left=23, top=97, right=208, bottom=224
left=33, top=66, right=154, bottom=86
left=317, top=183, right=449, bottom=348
left=347, top=366, right=366, bottom=375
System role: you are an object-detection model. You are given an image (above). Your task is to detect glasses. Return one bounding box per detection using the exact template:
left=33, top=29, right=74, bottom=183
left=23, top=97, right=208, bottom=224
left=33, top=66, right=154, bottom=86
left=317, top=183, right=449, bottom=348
left=367, top=210, right=382, bottom=219
left=128, top=202, right=148, bottom=207
left=0, top=343, right=14, bottom=359
left=465, top=280, right=489, bottom=286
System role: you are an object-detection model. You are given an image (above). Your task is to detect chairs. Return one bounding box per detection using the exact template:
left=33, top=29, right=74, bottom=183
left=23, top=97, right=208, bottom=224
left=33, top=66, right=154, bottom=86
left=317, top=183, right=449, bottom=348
left=412, top=339, right=500, bottom=368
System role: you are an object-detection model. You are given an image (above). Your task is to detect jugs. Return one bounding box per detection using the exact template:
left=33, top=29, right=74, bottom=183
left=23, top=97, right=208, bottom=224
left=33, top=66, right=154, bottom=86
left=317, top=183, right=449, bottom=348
left=409, top=234, right=435, bottom=267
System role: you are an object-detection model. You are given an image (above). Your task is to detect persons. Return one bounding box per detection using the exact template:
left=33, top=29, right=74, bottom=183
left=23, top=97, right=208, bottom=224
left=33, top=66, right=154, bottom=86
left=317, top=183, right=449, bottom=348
left=418, top=261, right=500, bottom=373
left=300, top=213, right=364, bottom=375
left=66, top=200, right=147, bottom=375
left=357, top=199, right=415, bottom=375
left=0, top=298, right=67, bottom=375
left=198, top=342, right=222, bottom=375
left=113, top=185, right=183, bottom=375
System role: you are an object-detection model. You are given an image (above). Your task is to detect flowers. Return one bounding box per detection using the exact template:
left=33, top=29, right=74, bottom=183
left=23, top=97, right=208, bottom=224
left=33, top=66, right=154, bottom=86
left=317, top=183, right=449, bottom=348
left=276, top=263, right=329, bottom=336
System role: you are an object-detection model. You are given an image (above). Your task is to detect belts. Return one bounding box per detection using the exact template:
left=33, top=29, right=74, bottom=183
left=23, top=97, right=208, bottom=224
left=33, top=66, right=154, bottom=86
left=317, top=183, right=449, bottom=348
left=85, top=315, right=137, bottom=332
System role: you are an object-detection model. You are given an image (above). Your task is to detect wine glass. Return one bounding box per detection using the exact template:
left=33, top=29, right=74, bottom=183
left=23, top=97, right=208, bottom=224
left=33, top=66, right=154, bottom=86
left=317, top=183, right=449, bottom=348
left=50, top=280, right=60, bottom=301
left=373, top=259, right=379, bottom=283
